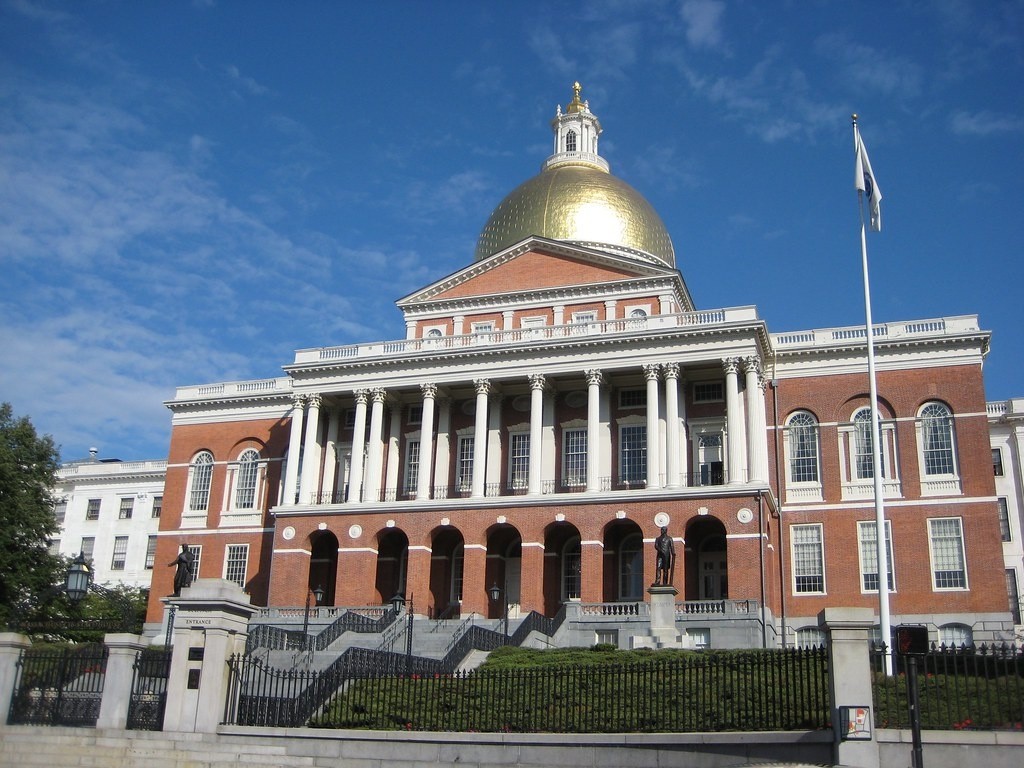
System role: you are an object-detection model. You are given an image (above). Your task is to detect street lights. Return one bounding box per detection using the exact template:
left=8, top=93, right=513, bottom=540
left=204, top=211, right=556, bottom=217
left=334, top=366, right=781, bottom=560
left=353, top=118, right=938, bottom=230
left=490, top=577, right=509, bottom=645
left=391, top=591, right=414, bottom=655
left=301, top=584, right=326, bottom=652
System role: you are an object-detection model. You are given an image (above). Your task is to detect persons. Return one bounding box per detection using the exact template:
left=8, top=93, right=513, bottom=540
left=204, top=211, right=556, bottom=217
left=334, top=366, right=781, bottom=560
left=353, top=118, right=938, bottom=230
left=651, top=526, right=676, bottom=586
left=167, top=543, right=195, bottom=597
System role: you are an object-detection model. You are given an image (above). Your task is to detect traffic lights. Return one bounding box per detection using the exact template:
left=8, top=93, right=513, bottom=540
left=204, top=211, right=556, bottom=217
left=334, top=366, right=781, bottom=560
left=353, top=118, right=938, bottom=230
left=895, top=624, right=929, bottom=657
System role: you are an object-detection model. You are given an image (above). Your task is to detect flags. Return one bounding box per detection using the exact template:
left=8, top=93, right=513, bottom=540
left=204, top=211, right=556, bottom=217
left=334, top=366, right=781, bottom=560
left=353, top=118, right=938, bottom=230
left=855, top=126, right=883, bottom=232
